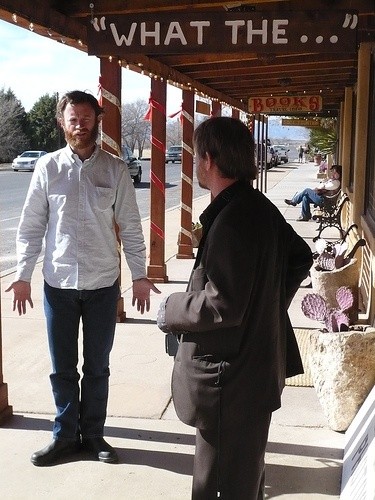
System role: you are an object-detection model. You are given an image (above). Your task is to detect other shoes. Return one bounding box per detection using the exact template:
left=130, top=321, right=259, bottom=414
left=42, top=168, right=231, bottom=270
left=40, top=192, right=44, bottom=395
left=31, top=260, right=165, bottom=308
left=296, top=217, right=309, bottom=221
left=284, top=199, right=297, bottom=206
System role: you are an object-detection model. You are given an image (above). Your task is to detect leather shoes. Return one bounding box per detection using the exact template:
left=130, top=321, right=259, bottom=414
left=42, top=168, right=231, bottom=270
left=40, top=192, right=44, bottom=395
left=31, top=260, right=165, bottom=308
left=32, top=438, right=81, bottom=466
left=82, top=438, right=118, bottom=462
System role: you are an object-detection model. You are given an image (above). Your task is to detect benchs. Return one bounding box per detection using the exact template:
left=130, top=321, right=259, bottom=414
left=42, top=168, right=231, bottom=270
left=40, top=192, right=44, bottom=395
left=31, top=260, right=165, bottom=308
left=313, top=188, right=366, bottom=259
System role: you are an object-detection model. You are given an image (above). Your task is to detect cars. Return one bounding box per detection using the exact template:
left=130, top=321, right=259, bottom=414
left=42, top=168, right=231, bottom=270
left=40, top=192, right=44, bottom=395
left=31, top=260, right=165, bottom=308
left=122, top=144, right=143, bottom=183
left=11, top=150, right=49, bottom=173
left=254, top=144, right=279, bottom=170
left=165, top=145, right=182, bottom=164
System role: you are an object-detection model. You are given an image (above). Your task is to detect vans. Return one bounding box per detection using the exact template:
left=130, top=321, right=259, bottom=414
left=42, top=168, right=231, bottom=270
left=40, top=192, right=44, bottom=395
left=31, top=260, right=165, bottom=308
left=273, top=145, right=290, bottom=164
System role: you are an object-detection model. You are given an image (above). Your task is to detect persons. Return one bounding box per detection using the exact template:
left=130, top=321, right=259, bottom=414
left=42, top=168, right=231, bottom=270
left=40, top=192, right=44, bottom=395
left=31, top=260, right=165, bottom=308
left=297, top=145, right=304, bottom=163
left=284, top=165, right=342, bottom=221
left=4, top=91, right=161, bottom=465
left=157, top=116, right=313, bottom=500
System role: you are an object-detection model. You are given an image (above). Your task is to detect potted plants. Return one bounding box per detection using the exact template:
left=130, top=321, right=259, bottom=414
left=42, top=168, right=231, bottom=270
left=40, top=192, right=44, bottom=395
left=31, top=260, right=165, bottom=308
left=309, top=239, right=359, bottom=326
left=301, top=286, right=375, bottom=431
